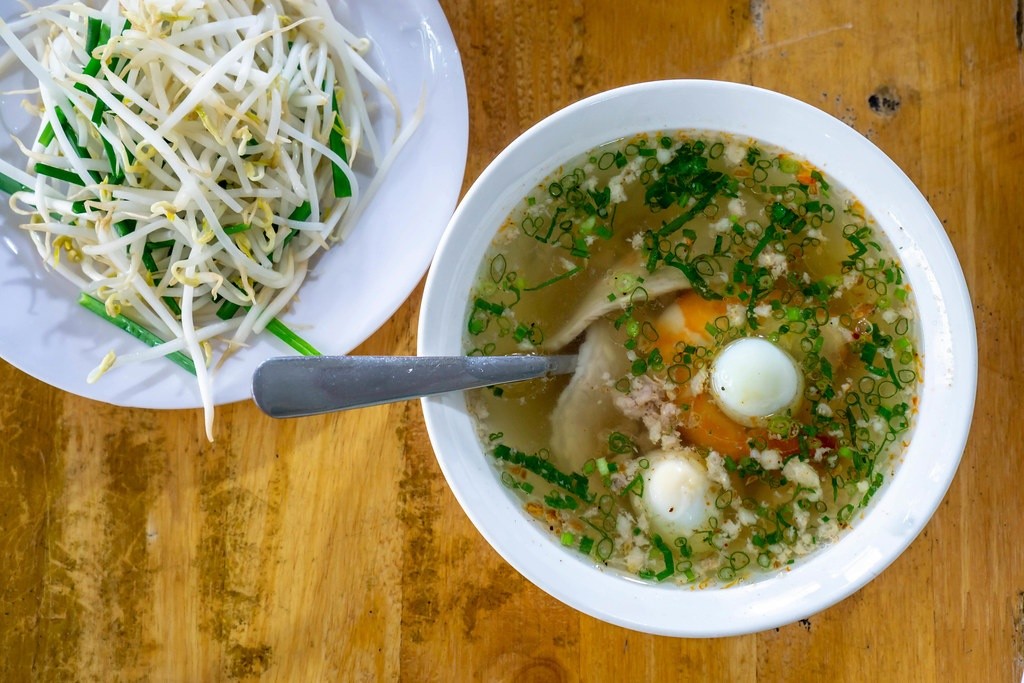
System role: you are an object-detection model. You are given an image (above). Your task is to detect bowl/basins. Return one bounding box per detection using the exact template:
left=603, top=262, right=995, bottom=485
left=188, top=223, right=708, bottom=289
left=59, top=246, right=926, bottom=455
left=416, top=78, right=979, bottom=639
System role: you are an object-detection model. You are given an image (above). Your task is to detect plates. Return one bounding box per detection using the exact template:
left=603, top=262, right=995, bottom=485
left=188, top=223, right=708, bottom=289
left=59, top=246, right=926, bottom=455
left=1, top=0, right=469, bottom=411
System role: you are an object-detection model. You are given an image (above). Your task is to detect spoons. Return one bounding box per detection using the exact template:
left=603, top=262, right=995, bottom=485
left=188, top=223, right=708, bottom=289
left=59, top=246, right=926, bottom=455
left=248, top=355, right=581, bottom=421
